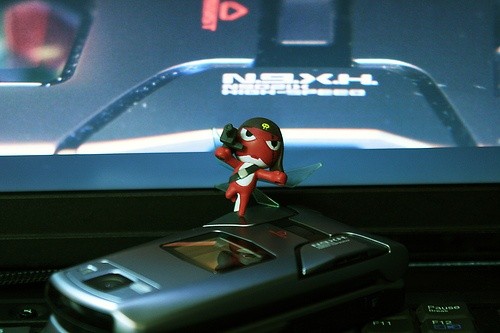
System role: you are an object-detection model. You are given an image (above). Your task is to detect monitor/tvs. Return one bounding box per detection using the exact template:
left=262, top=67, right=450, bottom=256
left=1, top=1, right=500, bottom=229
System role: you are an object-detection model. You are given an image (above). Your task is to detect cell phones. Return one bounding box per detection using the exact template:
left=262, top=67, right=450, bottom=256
left=42, top=203, right=410, bottom=333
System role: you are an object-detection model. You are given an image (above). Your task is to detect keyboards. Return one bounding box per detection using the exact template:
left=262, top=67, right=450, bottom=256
left=1, top=287, right=484, bottom=333
